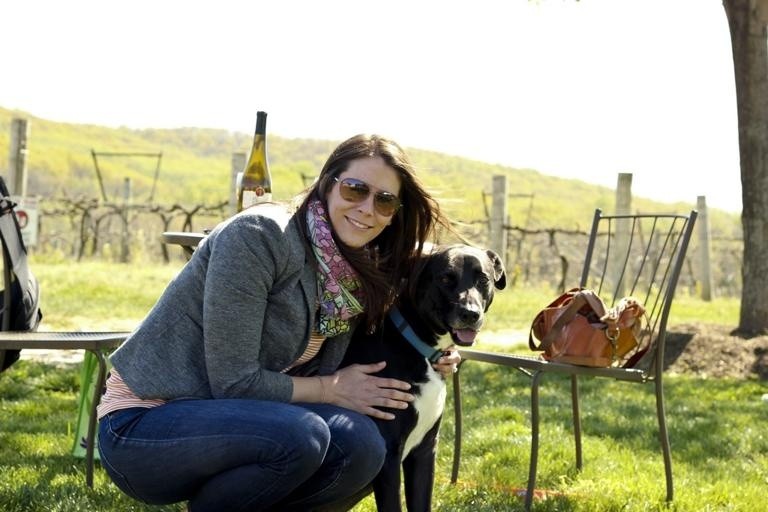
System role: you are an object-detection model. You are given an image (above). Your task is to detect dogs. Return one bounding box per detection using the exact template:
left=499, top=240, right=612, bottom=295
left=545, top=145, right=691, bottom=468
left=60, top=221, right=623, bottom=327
left=336, top=243, right=507, bottom=512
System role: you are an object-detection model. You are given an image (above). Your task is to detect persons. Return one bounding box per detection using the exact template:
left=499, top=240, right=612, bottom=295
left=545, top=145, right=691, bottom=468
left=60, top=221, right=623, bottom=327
left=98, top=134, right=462, bottom=512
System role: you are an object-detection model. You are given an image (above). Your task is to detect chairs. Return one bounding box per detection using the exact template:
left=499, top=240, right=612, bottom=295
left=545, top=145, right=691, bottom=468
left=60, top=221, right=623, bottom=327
left=450, top=208, right=699, bottom=512
left=0, top=239, right=133, bottom=491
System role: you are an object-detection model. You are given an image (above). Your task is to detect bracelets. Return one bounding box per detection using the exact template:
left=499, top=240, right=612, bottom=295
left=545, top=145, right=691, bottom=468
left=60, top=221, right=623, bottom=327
left=315, top=375, right=325, bottom=404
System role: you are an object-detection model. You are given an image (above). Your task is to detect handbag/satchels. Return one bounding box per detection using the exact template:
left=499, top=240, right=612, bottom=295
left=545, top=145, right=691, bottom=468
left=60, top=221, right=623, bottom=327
left=526, top=287, right=654, bottom=371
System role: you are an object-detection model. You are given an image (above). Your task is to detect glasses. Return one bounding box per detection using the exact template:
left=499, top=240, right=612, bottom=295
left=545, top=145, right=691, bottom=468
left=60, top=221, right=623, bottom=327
left=332, top=174, right=405, bottom=219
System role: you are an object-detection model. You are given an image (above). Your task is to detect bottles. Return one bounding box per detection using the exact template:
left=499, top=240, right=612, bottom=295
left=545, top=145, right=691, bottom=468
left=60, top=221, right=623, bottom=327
left=237, top=112, right=273, bottom=214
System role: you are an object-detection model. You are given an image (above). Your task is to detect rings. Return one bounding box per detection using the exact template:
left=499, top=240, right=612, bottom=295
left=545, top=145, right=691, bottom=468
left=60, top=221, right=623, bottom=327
left=453, top=366, right=459, bottom=373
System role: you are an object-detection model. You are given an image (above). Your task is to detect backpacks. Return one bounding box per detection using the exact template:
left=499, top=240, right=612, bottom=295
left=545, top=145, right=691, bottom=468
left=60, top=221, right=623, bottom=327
left=0, top=175, right=45, bottom=373
